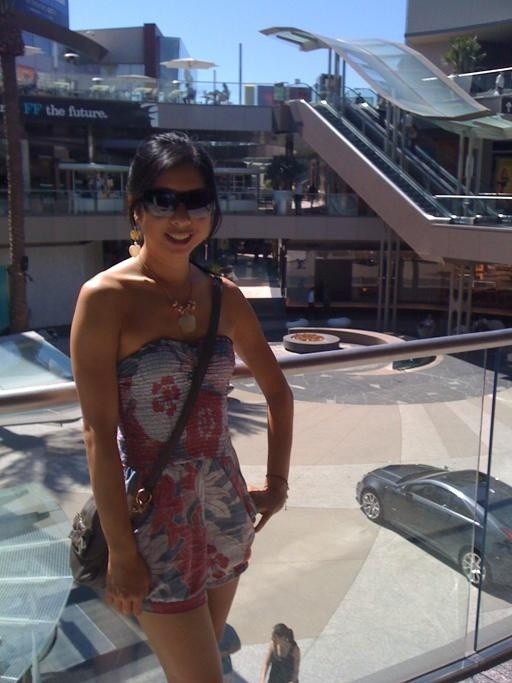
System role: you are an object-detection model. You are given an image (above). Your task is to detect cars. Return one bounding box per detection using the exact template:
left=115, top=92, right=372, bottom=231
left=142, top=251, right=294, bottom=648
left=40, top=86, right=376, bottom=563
left=356, top=461, right=512, bottom=600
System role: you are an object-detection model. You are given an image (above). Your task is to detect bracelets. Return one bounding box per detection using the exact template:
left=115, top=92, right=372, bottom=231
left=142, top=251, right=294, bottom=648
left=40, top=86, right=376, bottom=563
left=264, top=472, right=289, bottom=495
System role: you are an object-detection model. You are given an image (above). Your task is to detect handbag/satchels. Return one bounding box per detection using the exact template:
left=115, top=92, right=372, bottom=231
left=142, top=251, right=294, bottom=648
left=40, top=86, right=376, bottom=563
left=68, top=495, right=146, bottom=586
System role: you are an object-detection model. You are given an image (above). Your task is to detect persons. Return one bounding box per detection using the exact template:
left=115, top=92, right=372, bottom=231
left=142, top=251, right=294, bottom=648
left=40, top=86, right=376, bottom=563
left=68, top=129, right=298, bottom=682
left=308, top=182, right=317, bottom=208
left=356, top=91, right=364, bottom=103
left=494, top=71, right=508, bottom=95
left=218, top=621, right=241, bottom=682
left=259, top=621, right=301, bottom=683
left=183, top=82, right=195, bottom=104
left=293, top=177, right=304, bottom=215
left=306, top=285, right=315, bottom=311
left=215, top=82, right=229, bottom=104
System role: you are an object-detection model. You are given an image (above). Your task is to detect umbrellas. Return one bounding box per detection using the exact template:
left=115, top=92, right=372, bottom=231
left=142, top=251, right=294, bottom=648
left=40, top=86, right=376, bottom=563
left=159, top=56, right=219, bottom=79
left=117, top=73, right=155, bottom=89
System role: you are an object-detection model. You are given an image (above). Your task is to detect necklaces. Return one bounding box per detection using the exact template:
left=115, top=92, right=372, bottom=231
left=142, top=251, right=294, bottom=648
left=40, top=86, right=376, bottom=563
left=138, top=252, right=198, bottom=334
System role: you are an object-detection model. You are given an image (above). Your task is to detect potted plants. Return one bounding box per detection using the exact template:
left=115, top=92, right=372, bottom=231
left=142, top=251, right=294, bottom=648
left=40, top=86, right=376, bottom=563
left=440, top=32, right=489, bottom=100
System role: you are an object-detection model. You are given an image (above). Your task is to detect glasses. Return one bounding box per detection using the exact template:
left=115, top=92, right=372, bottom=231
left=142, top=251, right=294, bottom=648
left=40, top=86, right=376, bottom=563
left=139, top=186, right=215, bottom=220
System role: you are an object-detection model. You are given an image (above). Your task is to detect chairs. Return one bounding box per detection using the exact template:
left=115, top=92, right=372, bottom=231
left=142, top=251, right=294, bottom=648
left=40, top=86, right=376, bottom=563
left=41, top=82, right=230, bottom=105
